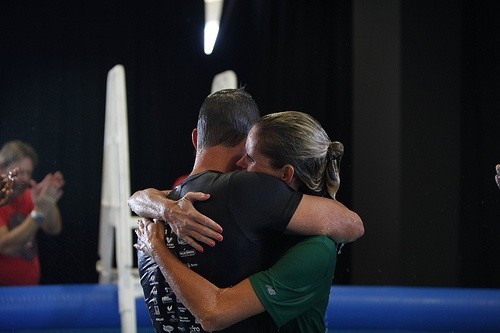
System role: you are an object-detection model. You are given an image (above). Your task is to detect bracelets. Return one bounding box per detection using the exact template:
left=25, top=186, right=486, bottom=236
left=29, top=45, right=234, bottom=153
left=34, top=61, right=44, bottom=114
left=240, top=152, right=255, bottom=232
left=29, top=210, right=45, bottom=225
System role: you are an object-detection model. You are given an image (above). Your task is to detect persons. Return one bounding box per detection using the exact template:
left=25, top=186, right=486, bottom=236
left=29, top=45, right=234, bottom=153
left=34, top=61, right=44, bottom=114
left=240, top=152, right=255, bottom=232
left=136, top=87, right=365, bottom=333
left=129, top=111, right=343, bottom=333
left=0, top=139, right=69, bottom=288
